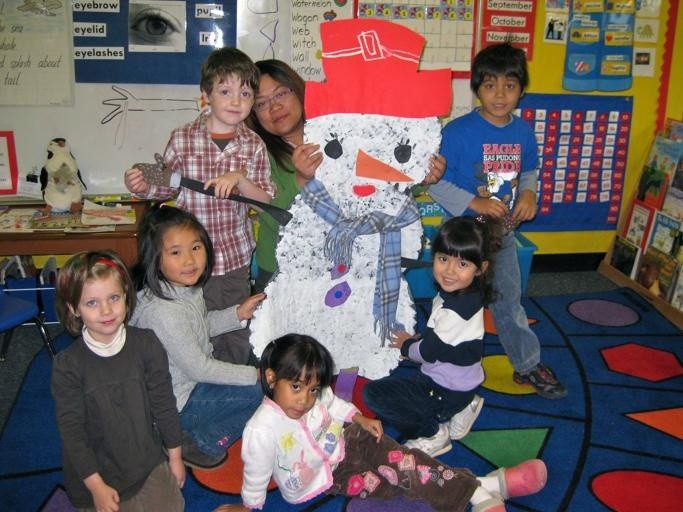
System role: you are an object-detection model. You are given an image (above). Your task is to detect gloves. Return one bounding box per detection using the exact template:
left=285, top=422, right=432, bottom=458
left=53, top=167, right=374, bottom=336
left=133, top=153, right=181, bottom=189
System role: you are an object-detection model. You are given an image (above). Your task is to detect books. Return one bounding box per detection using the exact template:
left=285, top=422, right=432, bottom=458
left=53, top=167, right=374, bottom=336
left=609, top=116, right=683, bottom=313
left=569, top=0, right=635, bottom=76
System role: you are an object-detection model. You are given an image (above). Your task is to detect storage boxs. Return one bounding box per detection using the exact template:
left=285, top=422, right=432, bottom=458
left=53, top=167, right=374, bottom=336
left=402, top=221, right=539, bottom=297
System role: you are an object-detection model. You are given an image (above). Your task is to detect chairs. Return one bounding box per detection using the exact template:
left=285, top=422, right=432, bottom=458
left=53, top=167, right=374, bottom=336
left=0, top=251, right=61, bottom=363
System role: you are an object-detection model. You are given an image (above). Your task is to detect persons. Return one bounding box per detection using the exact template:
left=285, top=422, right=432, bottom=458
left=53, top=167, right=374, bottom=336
left=128, top=0, right=186, bottom=46
left=244, top=59, right=323, bottom=296
left=115, top=45, right=276, bottom=308
left=362, top=213, right=493, bottom=458
left=209, top=333, right=548, bottom=512
left=425, top=44, right=567, bottom=396
left=51, top=248, right=186, bottom=508
left=127, top=204, right=269, bottom=464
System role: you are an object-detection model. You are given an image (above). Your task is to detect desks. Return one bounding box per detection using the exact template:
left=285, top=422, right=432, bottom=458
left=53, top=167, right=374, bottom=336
left=1, top=197, right=143, bottom=269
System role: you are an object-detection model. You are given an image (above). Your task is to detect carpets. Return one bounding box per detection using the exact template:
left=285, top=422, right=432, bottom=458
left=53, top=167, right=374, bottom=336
left=0, top=287, right=683, bottom=512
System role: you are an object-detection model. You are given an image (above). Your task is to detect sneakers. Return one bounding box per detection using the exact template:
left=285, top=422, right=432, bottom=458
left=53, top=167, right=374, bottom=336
left=513, top=363, right=567, bottom=399
left=163, top=434, right=227, bottom=470
left=403, top=394, right=484, bottom=458
left=472, top=459, right=548, bottom=512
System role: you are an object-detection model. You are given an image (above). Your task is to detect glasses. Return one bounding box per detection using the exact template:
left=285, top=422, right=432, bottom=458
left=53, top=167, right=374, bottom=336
left=253, top=88, right=292, bottom=109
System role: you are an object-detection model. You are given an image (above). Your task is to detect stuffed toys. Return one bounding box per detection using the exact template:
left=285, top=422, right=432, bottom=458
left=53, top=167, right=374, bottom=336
left=26, top=138, right=87, bottom=216
